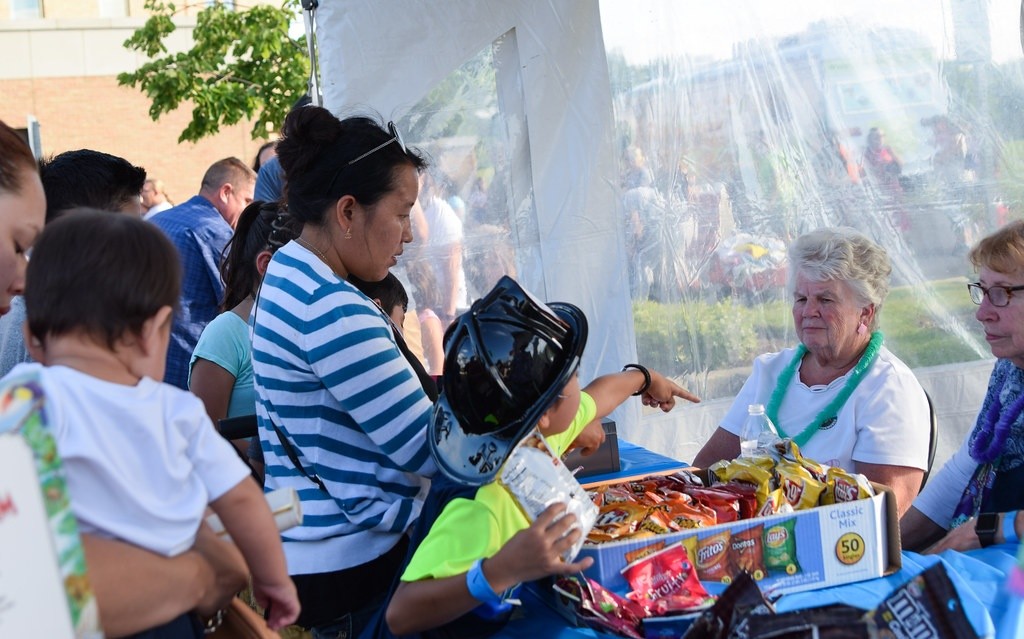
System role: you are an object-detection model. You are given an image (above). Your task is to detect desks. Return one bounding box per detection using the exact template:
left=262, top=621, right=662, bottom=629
left=482, top=439, right=691, bottom=639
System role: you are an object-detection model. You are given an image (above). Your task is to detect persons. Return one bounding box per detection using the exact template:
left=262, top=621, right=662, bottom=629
left=692, top=227, right=931, bottom=520
left=0, top=107, right=700, bottom=639
left=898, top=221, right=1024, bottom=556
left=647, top=114, right=1007, bottom=301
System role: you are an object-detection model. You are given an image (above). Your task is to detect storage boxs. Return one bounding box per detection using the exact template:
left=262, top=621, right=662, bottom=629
left=562, top=417, right=621, bottom=480
left=530, top=458, right=903, bottom=622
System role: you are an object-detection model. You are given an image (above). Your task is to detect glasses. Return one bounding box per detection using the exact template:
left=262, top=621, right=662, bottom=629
left=967, top=283, right=1024, bottom=308
left=349, top=120, right=407, bottom=165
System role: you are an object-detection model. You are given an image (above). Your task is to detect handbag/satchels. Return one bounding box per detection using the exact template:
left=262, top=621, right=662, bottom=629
left=202, top=595, right=282, bottom=639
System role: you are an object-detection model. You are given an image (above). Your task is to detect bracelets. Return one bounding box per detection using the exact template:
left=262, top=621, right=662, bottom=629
left=1003, top=510, right=1020, bottom=544
left=466, top=557, right=523, bottom=614
left=623, top=363, right=651, bottom=396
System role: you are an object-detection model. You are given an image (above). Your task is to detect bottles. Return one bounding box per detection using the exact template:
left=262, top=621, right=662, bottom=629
left=740, top=404, right=779, bottom=458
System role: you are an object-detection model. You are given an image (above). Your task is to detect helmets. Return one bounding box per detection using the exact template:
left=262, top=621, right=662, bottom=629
left=428, top=276, right=588, bottom=488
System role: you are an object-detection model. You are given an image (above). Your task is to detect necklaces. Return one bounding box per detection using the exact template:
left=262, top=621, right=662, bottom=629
left=971, top=372, right=1024, bottom=464
left=765, top=330, right=884, bottom=450
left=299, top=238, right=340, bottom=276
left=250, top=290, right=257, bottom=302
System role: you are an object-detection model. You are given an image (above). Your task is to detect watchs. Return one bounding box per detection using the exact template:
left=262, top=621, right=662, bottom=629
left=975, top=509, right=999, bottom=548
